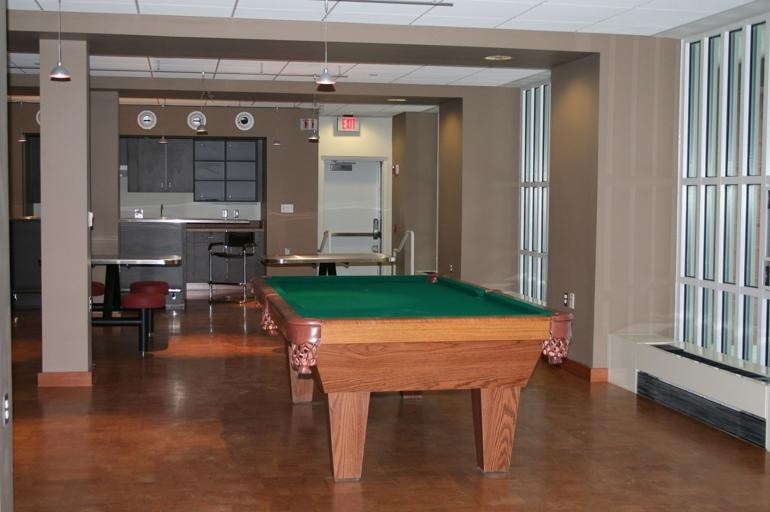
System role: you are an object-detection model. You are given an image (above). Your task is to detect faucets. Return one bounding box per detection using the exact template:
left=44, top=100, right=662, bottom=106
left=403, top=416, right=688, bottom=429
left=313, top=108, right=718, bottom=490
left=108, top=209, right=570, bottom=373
left=160, top=203, right=164, bottom=218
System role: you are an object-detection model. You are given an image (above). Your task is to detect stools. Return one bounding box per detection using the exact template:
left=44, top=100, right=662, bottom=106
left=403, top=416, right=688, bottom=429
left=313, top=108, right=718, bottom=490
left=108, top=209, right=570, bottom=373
left=124, top=293, right=165, bottom=353
left=91, top=280, right=104, bottom=306
left=131, top=273, right=169, bottom=333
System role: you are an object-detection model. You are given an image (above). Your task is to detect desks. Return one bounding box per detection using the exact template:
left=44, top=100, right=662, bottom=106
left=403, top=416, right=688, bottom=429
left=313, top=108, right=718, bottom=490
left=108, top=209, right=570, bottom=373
left=92, top=252, right=188, bottom=276
left=261, top=247, right=393, bottom=276
left=252, top=263, right=553, bottom=483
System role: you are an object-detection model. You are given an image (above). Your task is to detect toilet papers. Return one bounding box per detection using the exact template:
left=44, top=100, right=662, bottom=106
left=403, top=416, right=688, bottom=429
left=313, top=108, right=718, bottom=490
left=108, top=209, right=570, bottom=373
left=88, top=210, right=94, bottom=227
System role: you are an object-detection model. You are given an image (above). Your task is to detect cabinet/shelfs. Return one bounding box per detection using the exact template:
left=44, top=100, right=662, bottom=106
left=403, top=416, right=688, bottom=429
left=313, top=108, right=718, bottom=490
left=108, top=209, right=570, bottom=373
left=9, top=218, right=44, bottom=321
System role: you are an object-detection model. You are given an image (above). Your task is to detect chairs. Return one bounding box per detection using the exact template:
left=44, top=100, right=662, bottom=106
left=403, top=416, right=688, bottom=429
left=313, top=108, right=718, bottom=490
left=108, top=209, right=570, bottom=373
left=209, top=233, right=256, bottom=304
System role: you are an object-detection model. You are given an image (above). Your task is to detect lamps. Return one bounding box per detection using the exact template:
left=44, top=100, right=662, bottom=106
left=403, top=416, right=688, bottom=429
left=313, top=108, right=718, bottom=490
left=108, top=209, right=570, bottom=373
left=50, top=0, right=72, bottom=80
left=197, top=73, right=209, bottom=136
left=308, top=97, right=321, bottom=142
left=315, top=1, right=336, bottom=86
